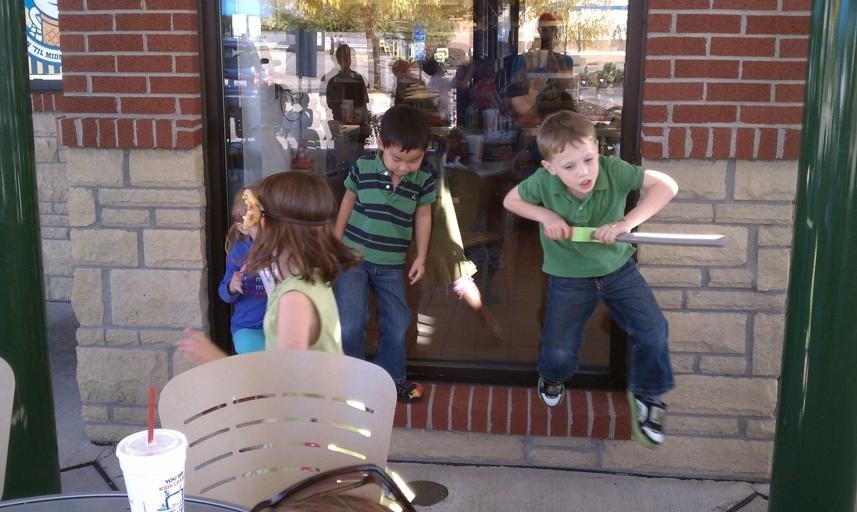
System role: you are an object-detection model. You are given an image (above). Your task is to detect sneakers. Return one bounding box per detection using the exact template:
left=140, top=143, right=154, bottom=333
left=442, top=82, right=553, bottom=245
left=537, top=375, right=565, bottom=409
left=627, top=390, right=667, bottom=449
left=396, top=381, right=426, bottom=401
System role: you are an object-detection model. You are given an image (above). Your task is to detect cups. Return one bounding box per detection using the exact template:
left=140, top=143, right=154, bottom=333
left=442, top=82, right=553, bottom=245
left=115, top=429, right=188, bottom=512
left=465, top=134, right=484, bottom=162
left=340, top=103, right=352, bottom=123
left=341, top=100, right=353, bottom=106
left=482, top=107, right=500, bottom=137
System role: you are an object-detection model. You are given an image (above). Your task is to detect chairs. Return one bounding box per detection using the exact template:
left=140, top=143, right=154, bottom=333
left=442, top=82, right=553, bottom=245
left=158, top=350, right=398, bottom=508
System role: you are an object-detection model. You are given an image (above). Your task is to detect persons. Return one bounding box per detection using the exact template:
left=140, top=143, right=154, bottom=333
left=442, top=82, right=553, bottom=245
left=260, top=8, right=582, bottom=194
left=501, top=106, right=679, bottom=451
left=214, top=181, right=272, bottom=357
left=408, top=214, right=506, bottom=345
left=169, top=169, right=418, bottom=511
left=329, top=103, right=437, bottom=403
